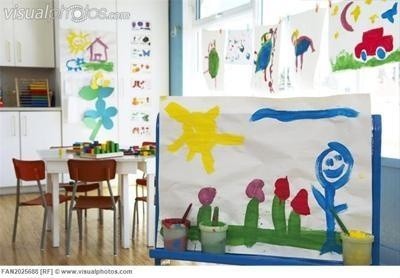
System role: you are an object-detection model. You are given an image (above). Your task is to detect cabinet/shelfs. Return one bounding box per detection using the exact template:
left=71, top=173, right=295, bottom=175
left=56, top=1, right=171, bottom=187
left=1, top=107, right=63, bottom=196
left=1, top=0, right=55, bottom=72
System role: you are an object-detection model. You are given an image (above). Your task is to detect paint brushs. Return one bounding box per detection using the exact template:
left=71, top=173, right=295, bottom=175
left=180, top=202, right=193, bottom=222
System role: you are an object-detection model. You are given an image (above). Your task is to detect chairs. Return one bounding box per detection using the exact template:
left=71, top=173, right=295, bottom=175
left=11, top=141, right=158, bottom=255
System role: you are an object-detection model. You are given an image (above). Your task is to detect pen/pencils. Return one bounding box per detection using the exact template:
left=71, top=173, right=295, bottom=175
left=210, top=207, right=219, bottom=226
left=330, top=210, right=350, bottom=237
